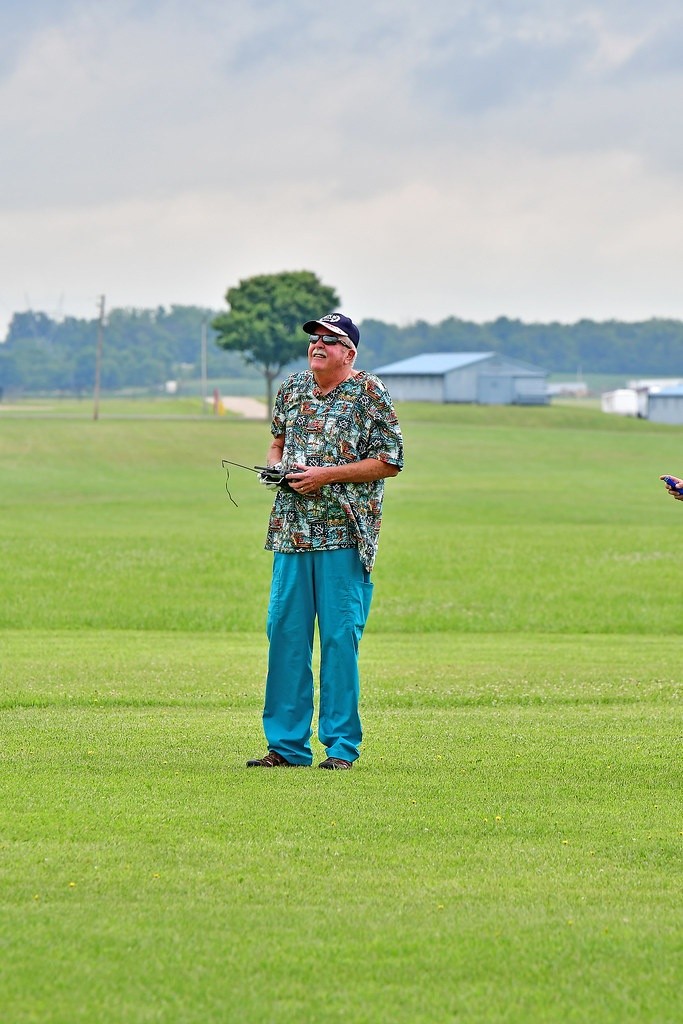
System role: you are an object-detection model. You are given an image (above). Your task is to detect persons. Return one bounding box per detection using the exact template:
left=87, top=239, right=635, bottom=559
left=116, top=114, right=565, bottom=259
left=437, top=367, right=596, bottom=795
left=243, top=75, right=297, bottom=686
left=660, top=475, right=683, bottom=501
left=246, top=313, right=404, bottom=770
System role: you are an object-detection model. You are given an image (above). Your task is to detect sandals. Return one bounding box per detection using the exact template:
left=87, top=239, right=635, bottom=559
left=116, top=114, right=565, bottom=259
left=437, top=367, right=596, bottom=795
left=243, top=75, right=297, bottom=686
left=246, top=750, right=301, bottom=767
left=318, top=757, right=352, bottom=770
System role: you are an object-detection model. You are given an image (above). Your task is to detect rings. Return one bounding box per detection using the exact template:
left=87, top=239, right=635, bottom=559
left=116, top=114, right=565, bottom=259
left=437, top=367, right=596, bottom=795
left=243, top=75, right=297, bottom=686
left=301, top=487, right=304, bottom=492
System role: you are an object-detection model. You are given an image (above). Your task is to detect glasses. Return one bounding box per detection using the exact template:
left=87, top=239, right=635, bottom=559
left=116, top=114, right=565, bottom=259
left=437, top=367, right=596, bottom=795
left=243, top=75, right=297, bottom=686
left=308, top=334, right=351, bottom=349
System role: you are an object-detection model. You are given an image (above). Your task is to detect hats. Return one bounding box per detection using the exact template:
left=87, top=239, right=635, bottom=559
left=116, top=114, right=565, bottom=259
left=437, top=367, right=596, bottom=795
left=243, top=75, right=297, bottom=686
left=302, top=312, right=359, bottom=349
